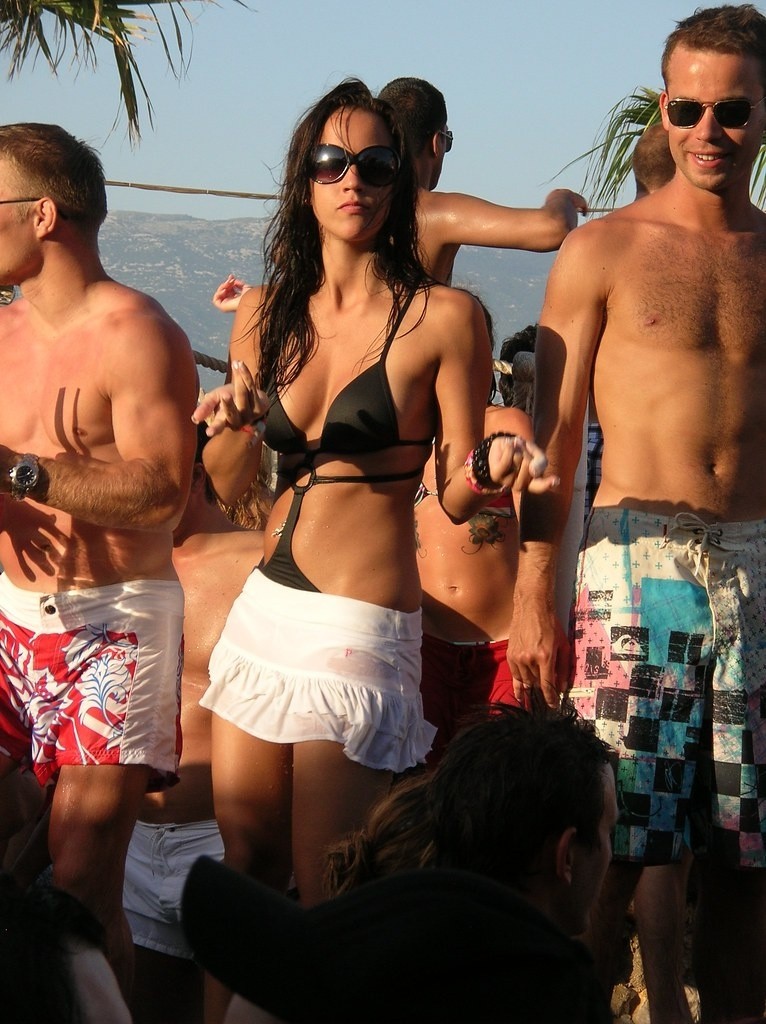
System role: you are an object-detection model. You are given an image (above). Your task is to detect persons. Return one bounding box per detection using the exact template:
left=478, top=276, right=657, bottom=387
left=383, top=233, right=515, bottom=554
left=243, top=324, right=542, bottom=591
left=0, top=4, right=766, bottom=1024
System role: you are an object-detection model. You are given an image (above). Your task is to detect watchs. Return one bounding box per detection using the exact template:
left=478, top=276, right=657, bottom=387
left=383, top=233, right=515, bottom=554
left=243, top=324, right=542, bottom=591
left=8, top=453, right=40, bottom=502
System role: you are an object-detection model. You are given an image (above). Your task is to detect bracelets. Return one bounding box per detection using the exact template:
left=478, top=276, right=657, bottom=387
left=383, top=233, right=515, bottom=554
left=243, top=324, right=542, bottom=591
left=242, top=411, right=269, bottom=448
left=464, top=450, right=505, bottom=497
left=473, top=432, right=518, bottom=488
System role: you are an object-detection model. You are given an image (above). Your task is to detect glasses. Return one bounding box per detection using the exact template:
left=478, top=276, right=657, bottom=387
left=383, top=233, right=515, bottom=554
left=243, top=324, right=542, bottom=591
left=435, top=129, right=453, bottom=153
left=664, top=97, right=766, bottom=128
left=301, top=142, right=407, bottom=187
